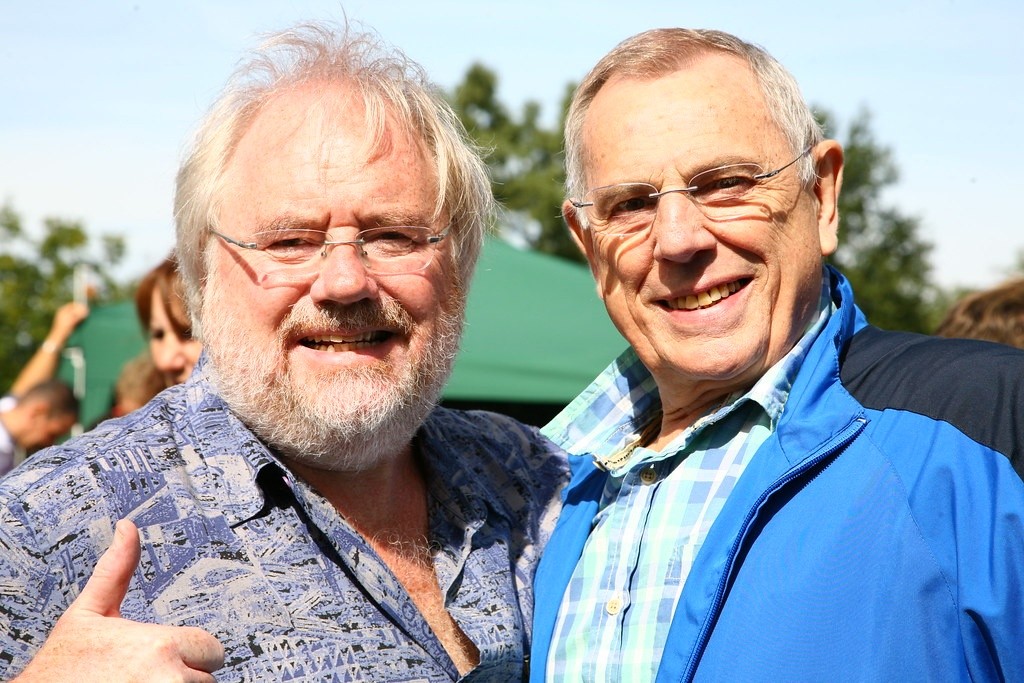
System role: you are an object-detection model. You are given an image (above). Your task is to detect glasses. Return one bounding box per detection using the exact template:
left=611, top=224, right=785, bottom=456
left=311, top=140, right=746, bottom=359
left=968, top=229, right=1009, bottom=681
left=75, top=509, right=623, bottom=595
left=208, top=218, right=461, bottom=279
left=565, top=145, right=811, bottom=223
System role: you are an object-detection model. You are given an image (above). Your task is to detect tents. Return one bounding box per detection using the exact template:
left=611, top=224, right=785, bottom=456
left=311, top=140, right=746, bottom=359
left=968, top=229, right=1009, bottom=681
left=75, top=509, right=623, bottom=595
left=55, top=226, right=631, bottom=420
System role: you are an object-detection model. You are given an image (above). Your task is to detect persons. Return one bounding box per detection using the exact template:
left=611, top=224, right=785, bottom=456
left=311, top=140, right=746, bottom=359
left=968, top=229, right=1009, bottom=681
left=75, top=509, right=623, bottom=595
left=0, top=0, right=569, bottom=683
left=526, top=28, right=1024, bottom=683
